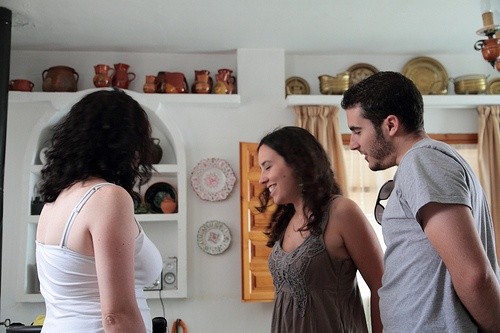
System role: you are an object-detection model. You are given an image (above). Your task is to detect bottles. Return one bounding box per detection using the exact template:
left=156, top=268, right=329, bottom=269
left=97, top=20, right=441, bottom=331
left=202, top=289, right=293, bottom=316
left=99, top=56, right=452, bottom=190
left=32, top=195, right=44, bottom=215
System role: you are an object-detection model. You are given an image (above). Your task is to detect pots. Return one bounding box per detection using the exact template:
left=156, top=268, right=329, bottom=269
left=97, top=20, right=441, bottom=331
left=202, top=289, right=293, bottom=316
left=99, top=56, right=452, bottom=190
left=41, top=65, right=80, bottom=92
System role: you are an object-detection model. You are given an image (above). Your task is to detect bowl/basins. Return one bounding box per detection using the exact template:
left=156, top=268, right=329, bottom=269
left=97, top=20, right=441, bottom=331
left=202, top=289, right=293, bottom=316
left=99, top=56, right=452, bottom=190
left=10, top=79, right=34, bottom=91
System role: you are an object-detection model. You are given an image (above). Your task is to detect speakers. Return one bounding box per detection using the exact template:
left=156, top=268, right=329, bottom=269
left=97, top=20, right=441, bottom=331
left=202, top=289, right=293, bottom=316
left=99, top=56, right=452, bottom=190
left=143, top=273, right=161, bottom=290
left=162, top=257, right=178, bottom=290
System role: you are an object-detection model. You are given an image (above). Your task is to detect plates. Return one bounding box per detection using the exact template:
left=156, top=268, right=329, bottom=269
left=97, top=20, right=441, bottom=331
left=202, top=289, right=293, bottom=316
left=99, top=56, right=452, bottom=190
left=196, top=220, right=232, bottom=255
left=144, top=181, right=177, bottom=213
left=400, top=56, right=449, bottom=94
left=285, top=76, right=309, bottom=95
left=190, top=157, right=237, bottom=202
left=347, top=63, right=379, bottom=88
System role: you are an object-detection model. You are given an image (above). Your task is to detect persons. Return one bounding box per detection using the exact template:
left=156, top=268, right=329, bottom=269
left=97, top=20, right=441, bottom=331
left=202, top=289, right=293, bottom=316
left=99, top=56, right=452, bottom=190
left=34, top=89, right=164, bottom=333
left=341, top=71, right=500, bottom=332
left=255, top=126, right=385, bottom=333
